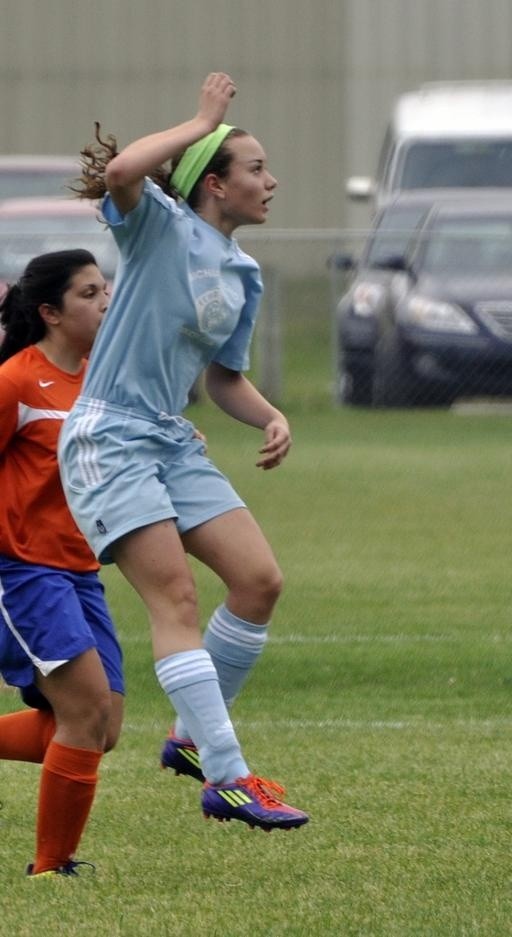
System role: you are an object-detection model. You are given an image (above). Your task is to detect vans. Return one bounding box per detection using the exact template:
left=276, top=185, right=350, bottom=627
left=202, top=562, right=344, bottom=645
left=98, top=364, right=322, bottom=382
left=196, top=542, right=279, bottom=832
left=343, top=81, right=512, bottom=210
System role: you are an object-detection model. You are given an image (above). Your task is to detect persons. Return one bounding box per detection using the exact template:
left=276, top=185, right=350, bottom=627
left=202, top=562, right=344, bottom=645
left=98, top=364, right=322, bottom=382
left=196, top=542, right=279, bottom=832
left=0, top=247, right=128, bottom=880
left=55, top=71, right=312, bottom=834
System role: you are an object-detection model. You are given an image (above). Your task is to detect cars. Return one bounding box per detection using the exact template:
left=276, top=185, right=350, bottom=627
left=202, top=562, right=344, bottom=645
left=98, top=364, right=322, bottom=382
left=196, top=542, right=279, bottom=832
left=0, top=155, right=120, bottom=336
left=325, top=186, right=512, bottom=406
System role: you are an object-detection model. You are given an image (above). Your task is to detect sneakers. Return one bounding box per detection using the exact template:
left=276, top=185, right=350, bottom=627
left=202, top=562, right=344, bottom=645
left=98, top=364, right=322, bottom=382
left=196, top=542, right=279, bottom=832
left=199, top=770, right=312, bottom=835
left=22, top=858, right=99, bottom=888
left=160, top=727, right=214, bottom=785
